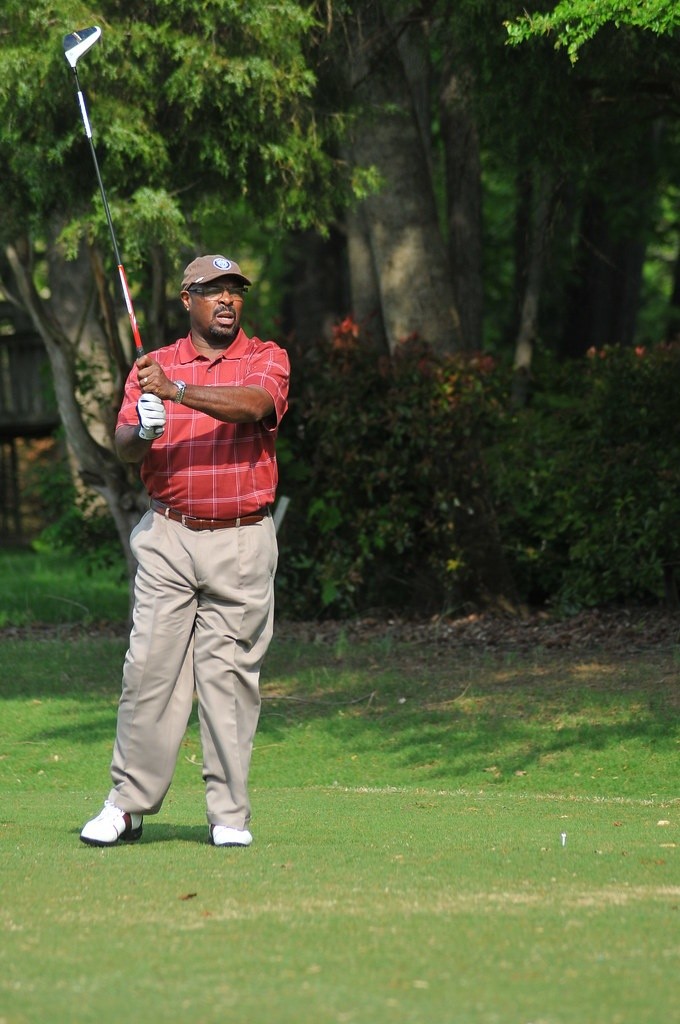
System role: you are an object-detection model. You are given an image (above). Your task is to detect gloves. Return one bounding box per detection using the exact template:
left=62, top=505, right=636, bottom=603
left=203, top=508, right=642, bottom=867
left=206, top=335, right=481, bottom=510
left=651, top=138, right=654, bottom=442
left=136, top=393, right=166, bottom=440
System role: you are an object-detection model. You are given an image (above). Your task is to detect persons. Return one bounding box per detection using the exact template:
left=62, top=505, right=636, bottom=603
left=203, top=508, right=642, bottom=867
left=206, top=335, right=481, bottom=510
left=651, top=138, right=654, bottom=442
left=77, top=253, right=290, bottom=845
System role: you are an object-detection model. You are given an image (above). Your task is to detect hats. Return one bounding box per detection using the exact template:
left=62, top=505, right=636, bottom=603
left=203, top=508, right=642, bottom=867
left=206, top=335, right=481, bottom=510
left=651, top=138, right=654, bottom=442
left=181, top=254, right=251, bottom=291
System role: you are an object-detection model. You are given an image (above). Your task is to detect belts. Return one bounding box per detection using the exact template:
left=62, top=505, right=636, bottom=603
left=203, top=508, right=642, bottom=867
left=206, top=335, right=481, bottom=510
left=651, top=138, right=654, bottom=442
left=149, top=500, right=272, bottom=531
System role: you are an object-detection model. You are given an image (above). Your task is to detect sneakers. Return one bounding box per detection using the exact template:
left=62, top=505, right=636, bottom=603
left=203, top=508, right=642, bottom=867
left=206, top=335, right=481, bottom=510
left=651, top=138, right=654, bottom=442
left=208, top=824, right=252, bottom=847
left=80, top=799, right=143, bottom=846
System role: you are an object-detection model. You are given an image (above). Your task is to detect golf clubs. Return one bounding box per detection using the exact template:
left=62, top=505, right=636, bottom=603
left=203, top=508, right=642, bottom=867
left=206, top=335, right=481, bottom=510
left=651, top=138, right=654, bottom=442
left=63, top=26, right=165, bottom=435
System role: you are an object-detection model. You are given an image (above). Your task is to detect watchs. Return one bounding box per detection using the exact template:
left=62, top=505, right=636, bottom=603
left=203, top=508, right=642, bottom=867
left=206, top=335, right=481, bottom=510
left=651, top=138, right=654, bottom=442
left=173, top=379, right=186, bottom=404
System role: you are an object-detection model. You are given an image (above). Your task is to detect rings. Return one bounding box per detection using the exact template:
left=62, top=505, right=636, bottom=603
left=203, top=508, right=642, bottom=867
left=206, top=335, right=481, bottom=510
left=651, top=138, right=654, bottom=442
left=144, top=377, right=148, bottom=384
